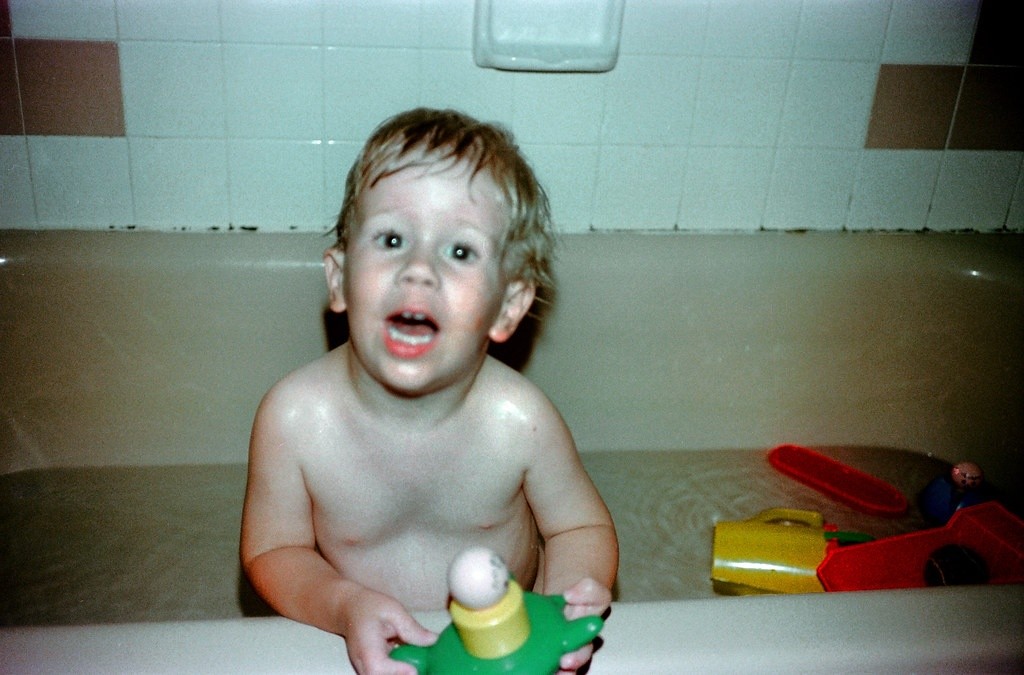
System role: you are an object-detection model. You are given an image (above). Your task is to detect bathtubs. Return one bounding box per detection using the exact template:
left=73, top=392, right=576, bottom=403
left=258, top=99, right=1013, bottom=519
left=0, top=228, right=1023, bottom=674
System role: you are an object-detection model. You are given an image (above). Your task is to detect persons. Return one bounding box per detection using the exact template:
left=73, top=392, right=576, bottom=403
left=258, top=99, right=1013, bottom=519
left=240, top=107, right=619, bottom=675
